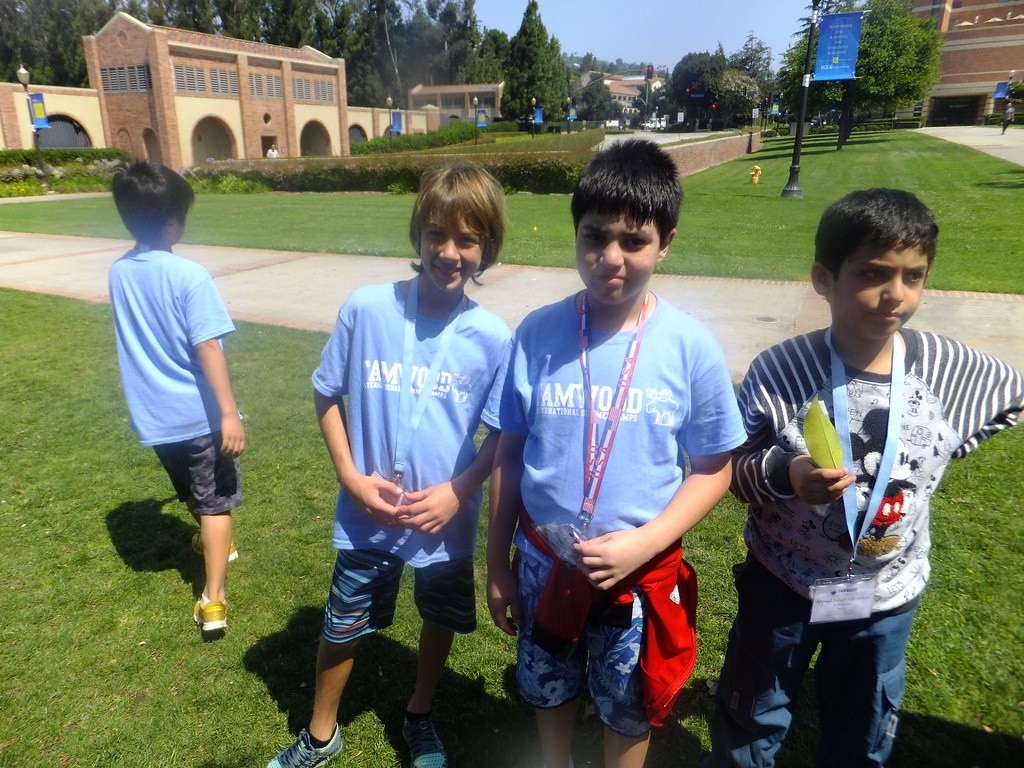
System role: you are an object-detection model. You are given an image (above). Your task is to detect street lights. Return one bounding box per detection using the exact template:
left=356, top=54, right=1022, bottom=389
left=386, top=96, right=393, bottom=157
left=532, top=97, right=536, bottom=139
left=567, top=97, right=570, bottom=133
left=472, top=96, right=478, bottom=144
left=1003, top=70, right=1015, bottom=110
left=16, top=64, right=50, bottom=191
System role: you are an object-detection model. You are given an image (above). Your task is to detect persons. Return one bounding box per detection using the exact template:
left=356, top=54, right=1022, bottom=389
left=266, top=163, right=514, bottom=768
left=998, top=102, right=1015, bottom=135
left=486, top=140, right=748, bottom=768
left=108, top=157, right=245, bottom=631
left=699, top=188, right=1024, bottom=768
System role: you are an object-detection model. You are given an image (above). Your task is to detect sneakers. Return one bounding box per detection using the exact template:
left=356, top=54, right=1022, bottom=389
left=402, top=717, right=448, bottom=768
left=194, top=597, right=227, bottom=631
left=190, top=529, right=238, bottom=563
left=267, top=723, right=343, bottom=768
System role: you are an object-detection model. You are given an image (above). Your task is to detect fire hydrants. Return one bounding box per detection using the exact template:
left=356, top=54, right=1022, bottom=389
left=750, top=166, right=761, bottom=183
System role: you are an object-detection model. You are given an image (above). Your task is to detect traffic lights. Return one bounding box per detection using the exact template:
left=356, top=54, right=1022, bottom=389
left=765, top=97, right=769, bottom=105
left=685, top=86, right=691, bottom=94
left=743, top=86, right=746, bottom=95
left=712, top=102, right=717, bottom=112
left=647, top=66, right=653, bottom=78
